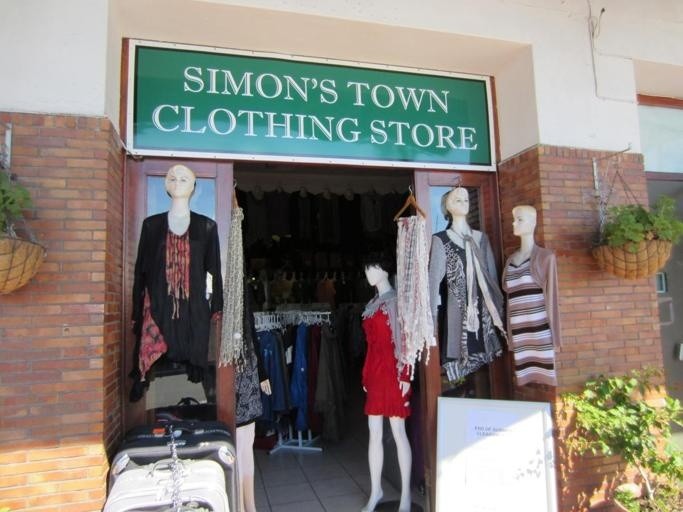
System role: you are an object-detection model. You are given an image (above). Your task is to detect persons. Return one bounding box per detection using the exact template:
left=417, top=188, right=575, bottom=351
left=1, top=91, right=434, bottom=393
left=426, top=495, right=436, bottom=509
left=360, top=250, right=413, bottom=512
left=130, top=165, right=223, bottom=365
left=234, top=286, right=273, bottom=512
left=501, top=204, right=562, bottom=386
left=427, top=185, right=505, bottom=384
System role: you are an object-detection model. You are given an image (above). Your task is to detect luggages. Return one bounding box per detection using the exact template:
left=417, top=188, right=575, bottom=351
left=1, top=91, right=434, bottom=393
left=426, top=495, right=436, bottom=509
left=103, top=421, right=237, bottom=512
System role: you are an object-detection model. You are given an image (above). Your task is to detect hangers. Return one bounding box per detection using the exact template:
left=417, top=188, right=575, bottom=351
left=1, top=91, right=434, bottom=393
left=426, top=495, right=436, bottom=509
left=252, top=310, right=284, bottom=333
left=392, top=183, right=429, bottom=222
left=294, top=310, right=333, bottom=327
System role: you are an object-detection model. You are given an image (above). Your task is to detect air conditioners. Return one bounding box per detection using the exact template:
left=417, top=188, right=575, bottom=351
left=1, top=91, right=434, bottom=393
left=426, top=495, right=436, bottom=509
left=658, top=296, right=674, bottom=326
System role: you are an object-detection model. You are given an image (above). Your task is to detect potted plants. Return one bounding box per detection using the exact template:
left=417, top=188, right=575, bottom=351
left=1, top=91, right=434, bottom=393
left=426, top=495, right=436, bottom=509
left=562, top=368, right=683, bottom=512
left=0, top=151, right=48, bottom=294
left=592, top=196, right=683, bottom=279
